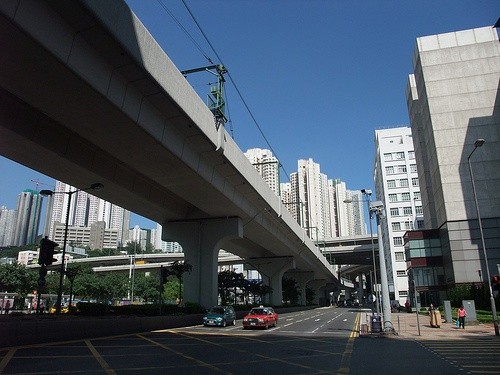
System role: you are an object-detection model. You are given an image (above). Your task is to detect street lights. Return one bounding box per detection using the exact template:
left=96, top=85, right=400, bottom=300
left=39, top=183, right=105, bottom=315
left=343, top=198, right=379, bottom=316
left=467, top=138, right=499, bottom=335
left=131, top=238, right=148, bottom=302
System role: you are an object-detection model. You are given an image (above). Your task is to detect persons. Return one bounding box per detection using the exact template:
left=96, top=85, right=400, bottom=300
left=34, top=300, right=37, bottom=309
left=397, top=302, right=400, bottom=312
left=55, top=302, right=64, bottom=306
left=5, top=300, right=10, bottom=314
left=392, top=301, right=395, bottom=308
left=40, top=303, right=44, bottom=314
left=458, top=306, right=466, bottom=329
left=405, top=301, right=409, bottom=312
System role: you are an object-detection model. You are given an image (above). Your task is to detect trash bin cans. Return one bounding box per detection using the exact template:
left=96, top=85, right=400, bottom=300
left=371, top=316, right=382, bottom=333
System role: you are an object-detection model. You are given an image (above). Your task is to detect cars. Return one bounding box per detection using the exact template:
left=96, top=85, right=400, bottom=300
left=202, top=305, right=237, bottom=328
left=242, top=305, right=279, bottom=330
left=48, top=304, right=70, bottom=314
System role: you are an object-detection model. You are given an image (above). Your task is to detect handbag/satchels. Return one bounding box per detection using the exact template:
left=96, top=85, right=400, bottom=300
left=456, top=320, right=459, bottom=326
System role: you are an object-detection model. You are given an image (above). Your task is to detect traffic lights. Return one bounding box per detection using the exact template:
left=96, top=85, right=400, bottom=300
left=37, top=238, right=59, bottom=265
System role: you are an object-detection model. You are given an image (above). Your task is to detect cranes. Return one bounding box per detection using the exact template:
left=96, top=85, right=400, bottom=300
left=25, top=177, right=49, bottom=192
